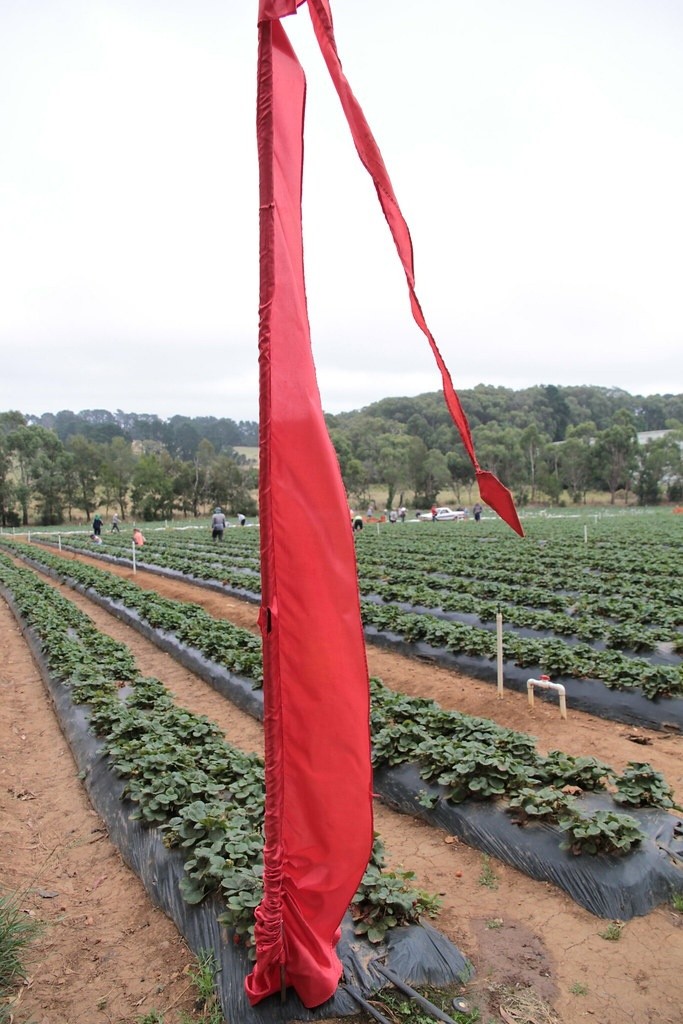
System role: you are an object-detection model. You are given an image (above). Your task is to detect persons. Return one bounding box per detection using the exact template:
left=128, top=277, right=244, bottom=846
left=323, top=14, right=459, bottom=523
left=88, top=498, right=483, bottom=554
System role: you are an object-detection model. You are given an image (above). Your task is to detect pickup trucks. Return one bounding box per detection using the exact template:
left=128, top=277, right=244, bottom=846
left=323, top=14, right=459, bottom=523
left=417, top=506, right=464, bottom=521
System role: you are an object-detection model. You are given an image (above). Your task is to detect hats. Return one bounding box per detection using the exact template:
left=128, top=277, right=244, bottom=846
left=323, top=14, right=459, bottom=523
left=214, top=507, right=222, bottom=512
